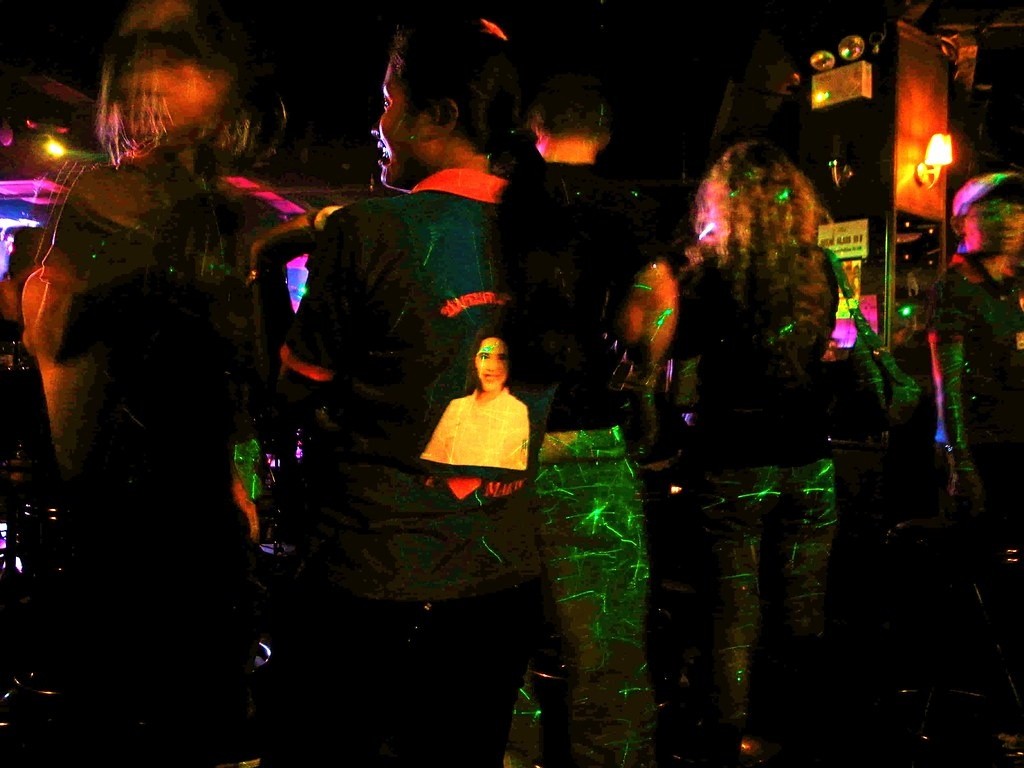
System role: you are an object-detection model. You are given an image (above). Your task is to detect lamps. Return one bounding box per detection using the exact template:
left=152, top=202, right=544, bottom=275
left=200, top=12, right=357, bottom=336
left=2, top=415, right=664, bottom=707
left=914, top=134, right=953, bottom=189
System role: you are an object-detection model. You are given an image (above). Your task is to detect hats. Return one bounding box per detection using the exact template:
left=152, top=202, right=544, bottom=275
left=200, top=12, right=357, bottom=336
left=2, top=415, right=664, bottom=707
left=952, top=171, right=1024, bottom=218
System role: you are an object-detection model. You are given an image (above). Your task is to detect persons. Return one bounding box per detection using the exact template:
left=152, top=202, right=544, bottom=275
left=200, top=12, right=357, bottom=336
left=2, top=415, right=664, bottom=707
left=421, top=325, right=529, bottom=472
left=0, top=0, right=1024, bottom=768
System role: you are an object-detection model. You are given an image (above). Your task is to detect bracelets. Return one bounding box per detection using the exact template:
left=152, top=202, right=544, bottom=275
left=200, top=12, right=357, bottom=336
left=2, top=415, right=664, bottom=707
left=951, top=459, right=974, bottom=472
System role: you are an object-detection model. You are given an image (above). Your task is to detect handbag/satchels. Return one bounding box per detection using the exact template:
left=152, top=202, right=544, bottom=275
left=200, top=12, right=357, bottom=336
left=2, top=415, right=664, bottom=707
left=820, top=247, right=922, bottom=440
